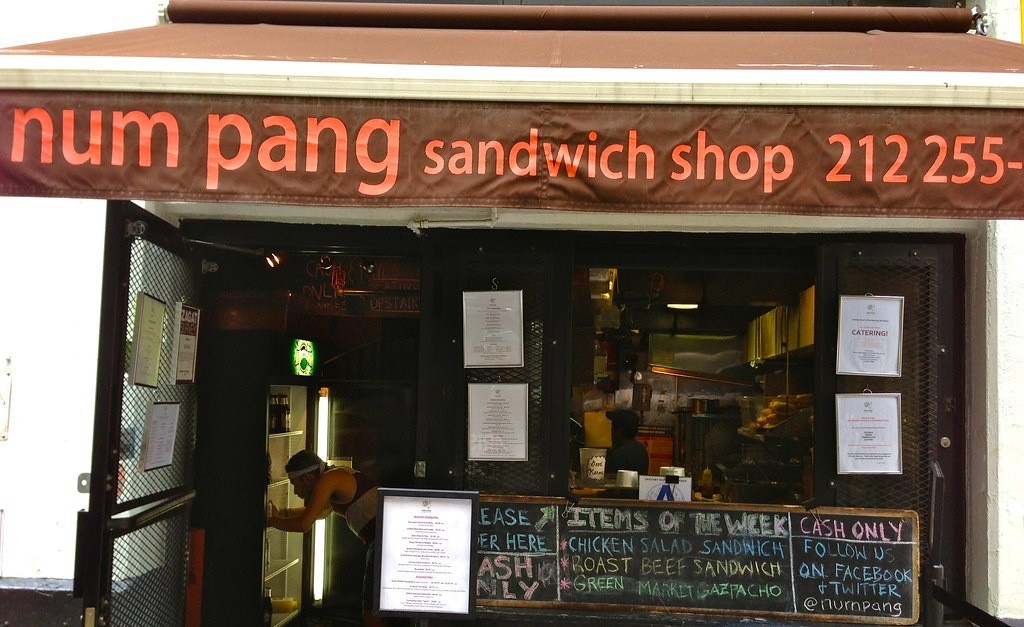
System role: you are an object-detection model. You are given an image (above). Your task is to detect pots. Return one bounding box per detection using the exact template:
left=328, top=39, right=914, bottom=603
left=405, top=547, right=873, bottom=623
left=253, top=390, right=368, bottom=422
left=702, top=399, right=720, bottom=413
left=691, top=398, right=702, bottom=414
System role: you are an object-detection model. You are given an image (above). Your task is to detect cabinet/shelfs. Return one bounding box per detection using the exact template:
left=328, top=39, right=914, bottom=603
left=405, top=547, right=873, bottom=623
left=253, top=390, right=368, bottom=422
left=264, top=386, right=308, bottom=627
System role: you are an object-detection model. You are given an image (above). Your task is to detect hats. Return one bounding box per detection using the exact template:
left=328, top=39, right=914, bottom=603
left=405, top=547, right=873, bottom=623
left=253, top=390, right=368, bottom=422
left=605, top=409, right=640, bottom=435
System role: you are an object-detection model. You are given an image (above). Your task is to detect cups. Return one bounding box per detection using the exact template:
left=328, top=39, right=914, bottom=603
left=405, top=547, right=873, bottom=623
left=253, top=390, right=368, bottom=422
left=579, top=448, right=605, bottom=484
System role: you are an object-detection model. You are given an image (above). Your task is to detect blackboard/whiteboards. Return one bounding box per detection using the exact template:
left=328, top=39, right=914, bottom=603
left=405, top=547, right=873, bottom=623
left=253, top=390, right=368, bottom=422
left=476, top=494, right=921, bottom=625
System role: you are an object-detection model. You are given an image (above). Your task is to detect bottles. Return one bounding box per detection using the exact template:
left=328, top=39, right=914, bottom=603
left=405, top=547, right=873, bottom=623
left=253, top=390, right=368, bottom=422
left=261, top=587, right=273, bottom=627
left=270, top=393, right=291, bottom=433
left=702, top=465, right=712, bottom=486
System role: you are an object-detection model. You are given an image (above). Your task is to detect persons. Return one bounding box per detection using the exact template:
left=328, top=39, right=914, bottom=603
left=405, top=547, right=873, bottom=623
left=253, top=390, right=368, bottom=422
left=605, top=409, right=649, bottom=498
left=268, top=450, right=387, bottom=627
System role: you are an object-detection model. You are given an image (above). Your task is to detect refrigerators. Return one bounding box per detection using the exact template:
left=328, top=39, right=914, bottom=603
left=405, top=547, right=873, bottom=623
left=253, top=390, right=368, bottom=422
left=184, top=375, right=314, bottom=627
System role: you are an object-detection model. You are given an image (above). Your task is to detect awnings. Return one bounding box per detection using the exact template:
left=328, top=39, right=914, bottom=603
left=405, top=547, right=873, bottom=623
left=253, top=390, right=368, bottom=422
left=0, top=0, right=1024, bottom=221
